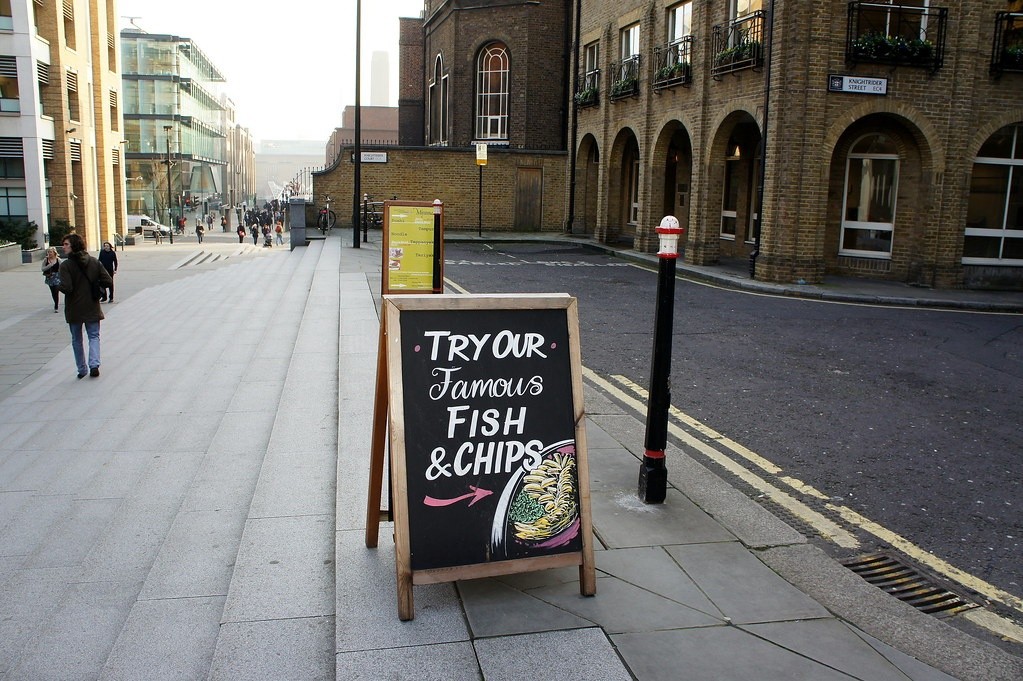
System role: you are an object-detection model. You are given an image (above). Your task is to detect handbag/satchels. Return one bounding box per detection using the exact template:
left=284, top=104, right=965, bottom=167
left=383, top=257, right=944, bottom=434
left=91, top=280, right=106, bottom=302
left=239, top=231, right=245, bottom=237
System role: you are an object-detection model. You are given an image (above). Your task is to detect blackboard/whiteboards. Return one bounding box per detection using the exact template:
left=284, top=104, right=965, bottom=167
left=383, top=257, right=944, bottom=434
left=365, top=292, right=595, bottom=584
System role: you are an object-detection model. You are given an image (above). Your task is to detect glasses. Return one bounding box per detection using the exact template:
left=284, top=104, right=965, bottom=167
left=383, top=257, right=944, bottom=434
left=63, top=242, right=74, bottom=248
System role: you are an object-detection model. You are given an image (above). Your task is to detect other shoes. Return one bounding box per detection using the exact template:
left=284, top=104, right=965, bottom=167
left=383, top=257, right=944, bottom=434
left=101, top=298, right=107, bottom=301
left=90, top=368, right=99, bottom=376
left=77, top=374, right=84, bottom=379
left=109, top=299, right=113, bottom=302
left=55, top=309, right=58, bottom=313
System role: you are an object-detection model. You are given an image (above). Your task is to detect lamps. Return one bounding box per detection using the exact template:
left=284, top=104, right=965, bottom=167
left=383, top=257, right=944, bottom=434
left=66, top=128, right=76, bottom=133
left=120, top=140, right=129, bottom=144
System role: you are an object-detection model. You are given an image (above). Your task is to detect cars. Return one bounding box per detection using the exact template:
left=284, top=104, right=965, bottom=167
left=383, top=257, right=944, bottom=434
left=127, top=214, right=170, bottom=237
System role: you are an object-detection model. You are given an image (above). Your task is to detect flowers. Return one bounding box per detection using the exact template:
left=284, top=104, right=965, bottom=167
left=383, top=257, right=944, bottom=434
left=852, top=30, right=933, bottom=61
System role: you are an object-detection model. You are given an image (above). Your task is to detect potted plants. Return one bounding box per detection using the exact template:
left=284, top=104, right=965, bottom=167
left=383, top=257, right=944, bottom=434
left=1004, top=45, right=1023, bottom=67
left=715, top=43, right=760, bottom=71
left=610, top=77, right=637, bottom=95
left=575, top=87, right=599, bottom=106
left=655, top=63, right=689, bottom=82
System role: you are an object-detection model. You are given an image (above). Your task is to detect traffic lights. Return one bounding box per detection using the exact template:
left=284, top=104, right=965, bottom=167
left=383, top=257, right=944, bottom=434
left=185, top=197, right=191, bottom=208
left=193, top=195, right=200, bottom=208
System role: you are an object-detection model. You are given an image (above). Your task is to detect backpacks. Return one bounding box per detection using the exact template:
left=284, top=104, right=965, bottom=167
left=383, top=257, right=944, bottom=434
left=222, top=219, right=227, bottom=225
left=276, top=226, right=281, bottom=232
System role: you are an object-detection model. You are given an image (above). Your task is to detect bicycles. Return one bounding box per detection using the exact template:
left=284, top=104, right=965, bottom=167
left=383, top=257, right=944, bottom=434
left=351, top=195, right=400, bottom=230
left=317, top=193, right=336, bottom=236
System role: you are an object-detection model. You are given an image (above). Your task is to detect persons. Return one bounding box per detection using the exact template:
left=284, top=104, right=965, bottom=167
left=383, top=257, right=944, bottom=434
left=178, top=217, right=187, bottom=234
left=245, top=200, right=286, bottom=227
left=41, top=247, right=61, bottom=313
left=262, top=223, right=271, bottom=237
left=98, top=242, right=118, bottom=303
left=207, top=215, right=214, bottom=230
left=54, top=234, right=113, bottom=379
left=196, top=223, right=204, bottom=244
left=185, top=197, right=190, bottom=206
left=275, top=222, right=284, bottom=246
left=220, top=216, right=227, bottom=233
left=251, top=224, right=258, bottom=245
left=236, top=223, right=246, bottom=243
